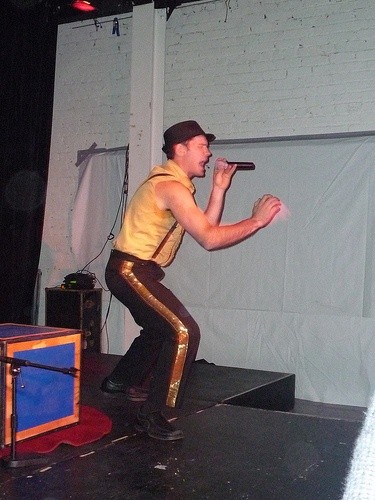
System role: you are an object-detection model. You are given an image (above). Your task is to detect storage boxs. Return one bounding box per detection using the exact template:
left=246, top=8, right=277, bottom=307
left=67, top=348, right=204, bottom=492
left=0, top=321, right=82, bottom=446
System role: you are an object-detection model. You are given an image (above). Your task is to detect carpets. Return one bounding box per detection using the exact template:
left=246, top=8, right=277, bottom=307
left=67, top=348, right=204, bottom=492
left=0, top=405, right=112, bottom=461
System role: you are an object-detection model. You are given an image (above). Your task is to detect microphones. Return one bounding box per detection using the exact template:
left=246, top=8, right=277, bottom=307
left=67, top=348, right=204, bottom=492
left=218, top=162, right=256, bottom=170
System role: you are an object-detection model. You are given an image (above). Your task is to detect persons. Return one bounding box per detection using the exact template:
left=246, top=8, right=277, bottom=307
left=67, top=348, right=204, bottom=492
left=99, top=120, right=282, bottom=440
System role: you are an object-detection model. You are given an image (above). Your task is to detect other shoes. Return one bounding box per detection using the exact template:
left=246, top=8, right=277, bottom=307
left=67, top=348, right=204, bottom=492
left=135, top=404, right=184, bottom=440
left=101, top=377, right=148, bottom=402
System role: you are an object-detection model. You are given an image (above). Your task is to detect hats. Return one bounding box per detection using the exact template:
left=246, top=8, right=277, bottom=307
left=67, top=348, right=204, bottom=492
left=162, top=120, right=216, bottom=152
